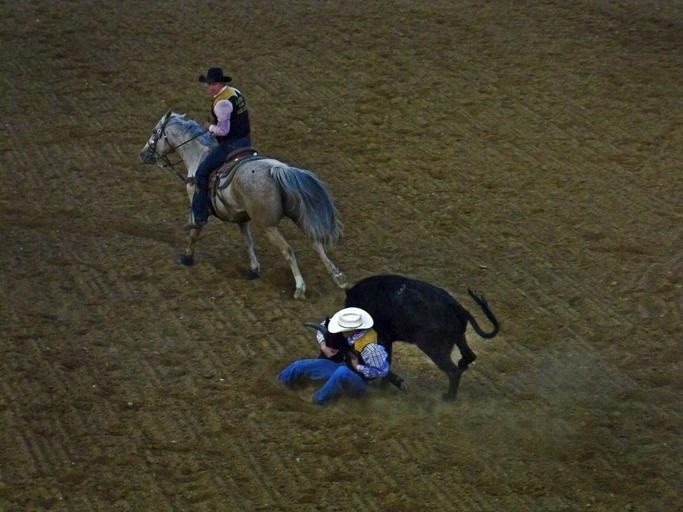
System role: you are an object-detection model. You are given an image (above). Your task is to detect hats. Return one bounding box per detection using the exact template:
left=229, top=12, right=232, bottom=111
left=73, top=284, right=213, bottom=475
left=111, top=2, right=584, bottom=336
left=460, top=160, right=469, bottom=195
left=327, top=307, right=374, bottom=334
left=199, top=68, right=232, bottom=83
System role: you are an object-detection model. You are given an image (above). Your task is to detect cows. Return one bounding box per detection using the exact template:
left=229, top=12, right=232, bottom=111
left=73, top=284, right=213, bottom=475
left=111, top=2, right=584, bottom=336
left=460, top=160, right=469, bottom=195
left=302, top=274, right=500, bottom=401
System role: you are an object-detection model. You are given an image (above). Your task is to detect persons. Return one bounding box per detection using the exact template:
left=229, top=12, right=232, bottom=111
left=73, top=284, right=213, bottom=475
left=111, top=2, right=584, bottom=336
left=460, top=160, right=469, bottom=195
left=276, top=307, right=390, bottom=405
left=183, top=68, right=251, bottom=230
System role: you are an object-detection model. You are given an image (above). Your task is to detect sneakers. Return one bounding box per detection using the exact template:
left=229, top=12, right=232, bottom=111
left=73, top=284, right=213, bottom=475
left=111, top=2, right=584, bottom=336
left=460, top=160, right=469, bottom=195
left=184, top=218, right=207, bottom=230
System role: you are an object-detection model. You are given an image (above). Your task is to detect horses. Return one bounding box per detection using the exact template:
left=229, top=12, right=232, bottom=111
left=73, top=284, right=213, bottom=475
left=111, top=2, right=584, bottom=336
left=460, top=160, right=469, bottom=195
left=138, top=107, right=357, bottom=301
